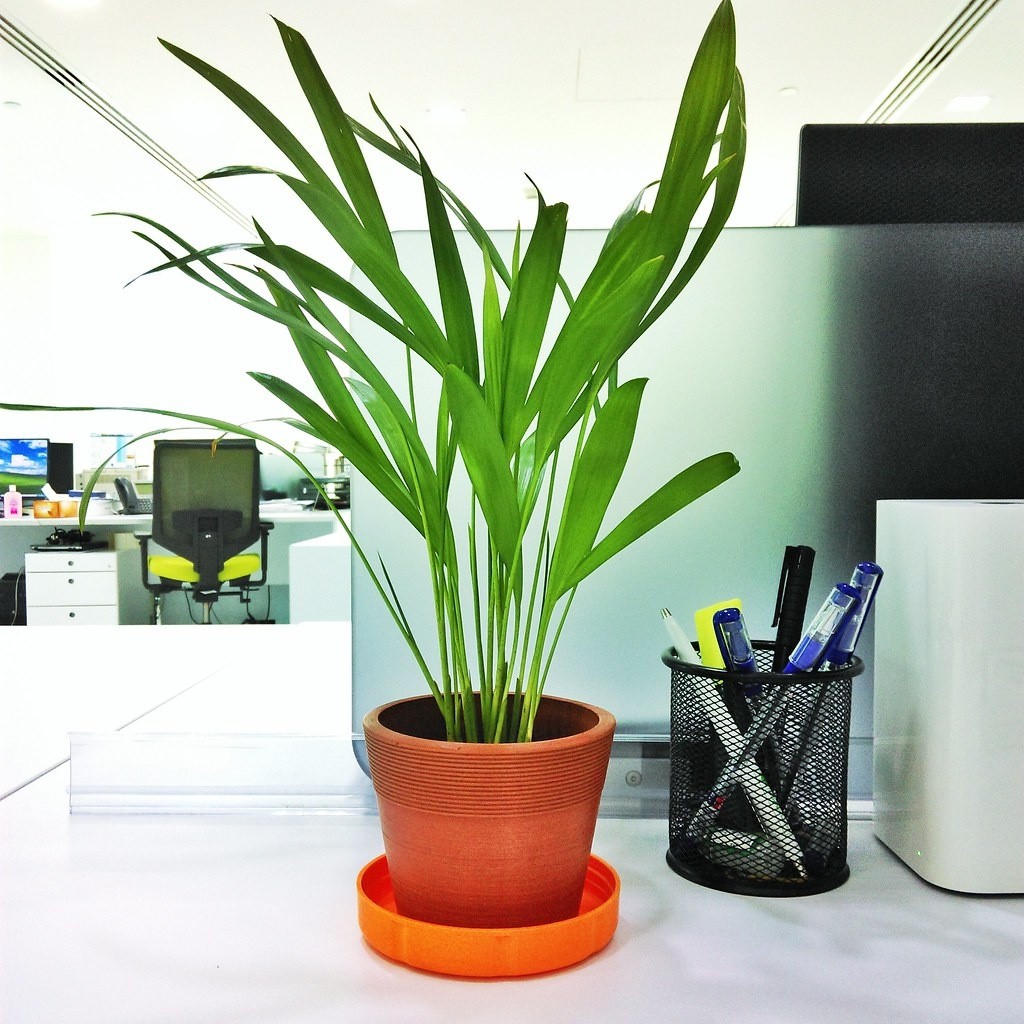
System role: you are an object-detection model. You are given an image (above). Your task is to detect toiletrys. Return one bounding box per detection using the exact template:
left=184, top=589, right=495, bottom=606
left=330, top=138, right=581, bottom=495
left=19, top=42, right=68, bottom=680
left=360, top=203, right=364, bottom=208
left=4, top=484, right=22, bottom=518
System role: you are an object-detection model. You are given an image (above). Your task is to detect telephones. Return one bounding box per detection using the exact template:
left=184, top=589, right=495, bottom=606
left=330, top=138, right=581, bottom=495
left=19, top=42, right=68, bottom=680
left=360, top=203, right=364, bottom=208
left=114, top=476, right=154, bottom=515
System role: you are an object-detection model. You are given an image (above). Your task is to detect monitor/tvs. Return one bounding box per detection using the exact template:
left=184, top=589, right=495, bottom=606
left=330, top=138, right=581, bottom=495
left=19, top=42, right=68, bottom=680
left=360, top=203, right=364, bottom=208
left=796, top=122, right=1024, bottom=228
left=0, top=438, right=74, bottom=517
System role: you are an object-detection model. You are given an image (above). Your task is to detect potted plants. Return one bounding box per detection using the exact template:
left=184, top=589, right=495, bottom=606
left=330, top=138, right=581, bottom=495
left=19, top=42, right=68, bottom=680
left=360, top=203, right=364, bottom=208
left=92, top=0, right=747, bottom=982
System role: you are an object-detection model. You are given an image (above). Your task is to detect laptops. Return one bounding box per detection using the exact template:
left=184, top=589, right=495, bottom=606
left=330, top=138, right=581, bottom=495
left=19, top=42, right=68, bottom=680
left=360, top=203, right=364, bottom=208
left=31, top=542, right=107, bottom=551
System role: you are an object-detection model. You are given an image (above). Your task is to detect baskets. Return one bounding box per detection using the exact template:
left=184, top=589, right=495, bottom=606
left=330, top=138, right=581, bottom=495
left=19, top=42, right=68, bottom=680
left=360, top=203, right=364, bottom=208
left=662, top=639, right=866, bottom=898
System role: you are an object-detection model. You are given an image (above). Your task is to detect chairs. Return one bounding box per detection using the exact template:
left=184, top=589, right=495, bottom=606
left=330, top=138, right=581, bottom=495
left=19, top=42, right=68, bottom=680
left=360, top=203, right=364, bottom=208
left=130, top=437, right=274, bottom=625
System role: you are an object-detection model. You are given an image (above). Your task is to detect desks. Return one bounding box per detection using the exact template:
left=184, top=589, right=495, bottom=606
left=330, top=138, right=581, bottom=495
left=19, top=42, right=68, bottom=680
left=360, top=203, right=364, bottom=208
left=0, top=496, right=336, bottom=626
left=0, top=624, right=1024, bottom=1023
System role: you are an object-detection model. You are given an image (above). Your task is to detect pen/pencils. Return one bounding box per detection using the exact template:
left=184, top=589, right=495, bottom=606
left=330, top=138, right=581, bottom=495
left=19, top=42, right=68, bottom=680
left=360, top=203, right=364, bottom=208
left=655, top=545, right=888, bottom=893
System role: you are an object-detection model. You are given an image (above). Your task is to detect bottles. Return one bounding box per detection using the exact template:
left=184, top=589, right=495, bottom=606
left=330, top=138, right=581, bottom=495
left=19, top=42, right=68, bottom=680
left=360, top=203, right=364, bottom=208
left=4, top=484, right=22, bottom=517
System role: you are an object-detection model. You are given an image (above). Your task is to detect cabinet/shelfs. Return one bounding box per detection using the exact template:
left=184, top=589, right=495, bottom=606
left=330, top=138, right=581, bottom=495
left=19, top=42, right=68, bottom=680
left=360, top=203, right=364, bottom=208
left=24, top=547, right=145, bottom=626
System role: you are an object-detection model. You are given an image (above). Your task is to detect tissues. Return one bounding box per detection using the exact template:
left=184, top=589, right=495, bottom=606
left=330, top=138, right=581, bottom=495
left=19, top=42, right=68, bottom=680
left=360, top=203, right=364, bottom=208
left=33, top=483, right=79, bottom=518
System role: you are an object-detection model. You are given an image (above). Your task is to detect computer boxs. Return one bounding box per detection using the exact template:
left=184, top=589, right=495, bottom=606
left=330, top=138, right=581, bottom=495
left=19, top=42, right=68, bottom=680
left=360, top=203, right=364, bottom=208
left=0, top=572, right=27, bottom=626
